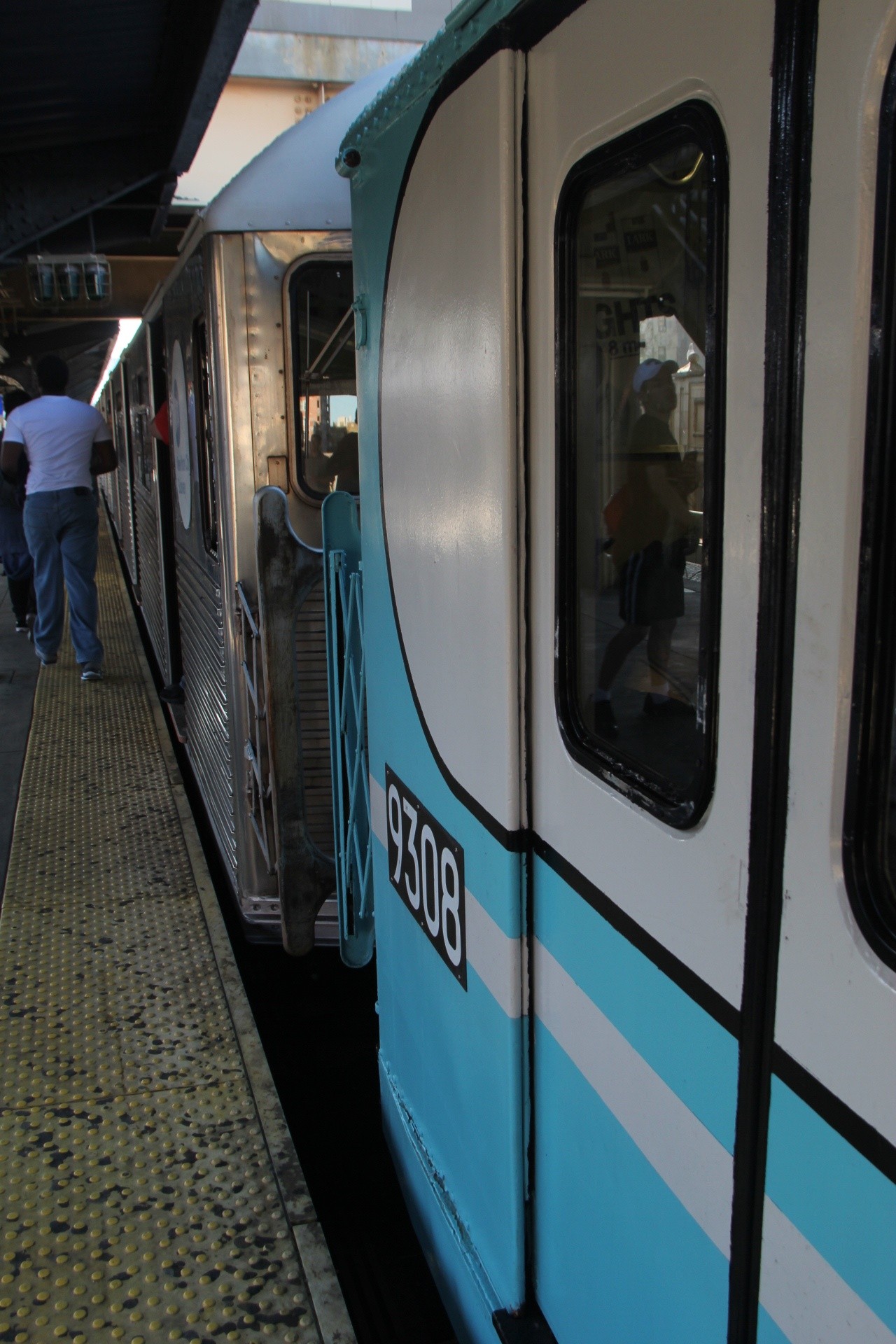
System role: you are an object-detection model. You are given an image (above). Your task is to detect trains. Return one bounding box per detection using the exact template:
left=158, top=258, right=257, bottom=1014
left=90, top=1, right=894, bottom=1344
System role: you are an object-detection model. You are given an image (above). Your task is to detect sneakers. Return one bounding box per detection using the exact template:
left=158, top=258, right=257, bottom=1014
left=81, top=661, right=102, bottom=680
left=34, top=643, right=59, bottom=667
left=26, top=614, right=37, bottom=643
left=15, top=620, right=30, bottom=632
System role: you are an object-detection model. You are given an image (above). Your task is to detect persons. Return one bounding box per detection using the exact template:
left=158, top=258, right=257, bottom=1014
left=0, top=355, right=120, bottom=680
left=305, top=409, right=361, bottom=496
left=148, top=396, right=169, bottom=447
left=588, top=359, right=704, bottom=714
